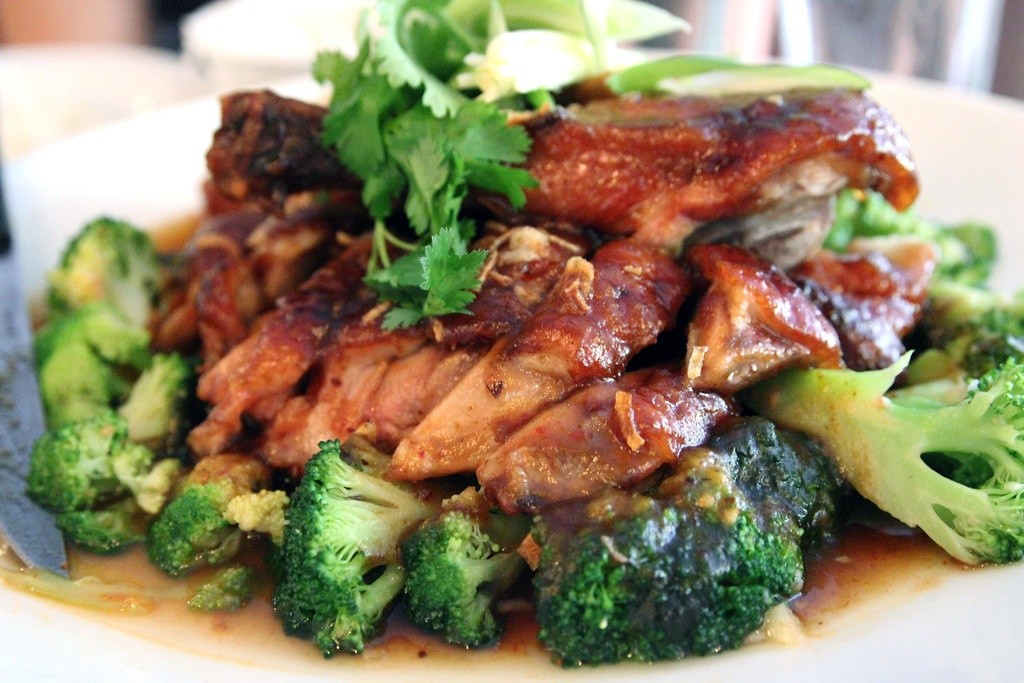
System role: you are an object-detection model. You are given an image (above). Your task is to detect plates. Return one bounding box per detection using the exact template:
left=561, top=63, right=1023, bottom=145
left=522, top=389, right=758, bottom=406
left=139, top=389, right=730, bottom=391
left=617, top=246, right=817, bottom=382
left=0, top=59, right=1024, bottom=681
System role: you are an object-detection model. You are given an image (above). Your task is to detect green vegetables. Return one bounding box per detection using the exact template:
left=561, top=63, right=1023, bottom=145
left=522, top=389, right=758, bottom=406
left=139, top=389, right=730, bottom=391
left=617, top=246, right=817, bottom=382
left=311, top=0, right=872, bottom=337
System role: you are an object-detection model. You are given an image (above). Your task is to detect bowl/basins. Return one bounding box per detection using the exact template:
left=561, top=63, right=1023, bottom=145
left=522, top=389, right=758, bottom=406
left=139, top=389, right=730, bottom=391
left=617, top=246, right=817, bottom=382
left=0, top=0, right=368, bottom=148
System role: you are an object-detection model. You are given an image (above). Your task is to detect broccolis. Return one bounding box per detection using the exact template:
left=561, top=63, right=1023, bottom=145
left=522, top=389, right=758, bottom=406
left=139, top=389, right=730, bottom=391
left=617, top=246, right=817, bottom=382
left=34, top=212, right=1024, bottom=668
